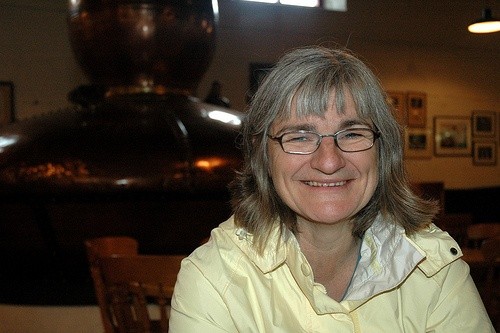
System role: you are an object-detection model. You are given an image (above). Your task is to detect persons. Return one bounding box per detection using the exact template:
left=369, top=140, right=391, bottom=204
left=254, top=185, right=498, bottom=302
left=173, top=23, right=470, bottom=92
left=169, top=46, right=498, bottom=333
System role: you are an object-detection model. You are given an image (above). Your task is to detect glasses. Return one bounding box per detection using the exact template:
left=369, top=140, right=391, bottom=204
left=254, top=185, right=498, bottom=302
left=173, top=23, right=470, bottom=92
left=266, top=128, right=380, bottom=155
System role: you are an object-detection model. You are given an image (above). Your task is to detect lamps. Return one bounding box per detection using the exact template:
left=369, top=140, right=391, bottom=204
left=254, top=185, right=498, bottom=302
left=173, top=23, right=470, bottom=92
left=466, top=0, right=500, bottom=34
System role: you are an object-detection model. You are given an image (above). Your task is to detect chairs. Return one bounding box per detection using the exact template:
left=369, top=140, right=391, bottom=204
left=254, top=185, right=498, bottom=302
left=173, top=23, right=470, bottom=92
left=460, top=221, right=500, bottom=307
left=87, top=233, right=209, bottom=333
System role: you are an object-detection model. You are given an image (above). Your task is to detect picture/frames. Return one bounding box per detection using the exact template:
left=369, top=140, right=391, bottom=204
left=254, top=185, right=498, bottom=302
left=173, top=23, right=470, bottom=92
left=404, top=128, right=432, bottom=161
left=407, top=91, right=428, bottom=129
left=433, top=117, right=472, bottom=158
left=385, top=92, right=405, bottom=126
left=471, top=110, right=497, bottom=139
left=472, top=140, right=497, bottom=166
